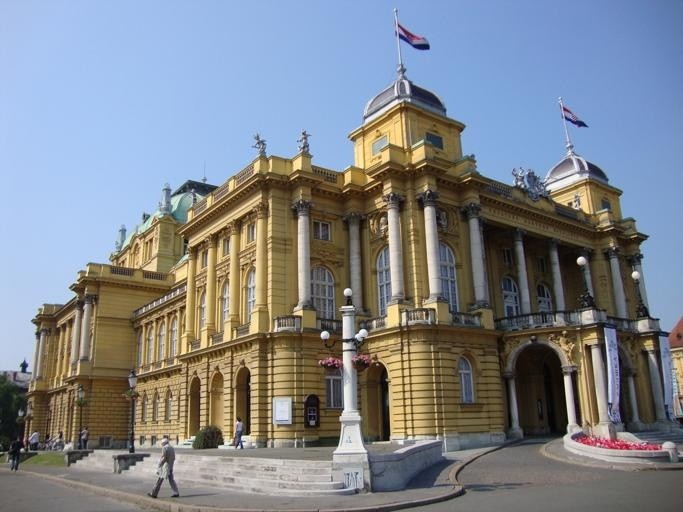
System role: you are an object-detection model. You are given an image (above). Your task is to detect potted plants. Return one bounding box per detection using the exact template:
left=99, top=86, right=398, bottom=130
left=121, top=390, right=140, bottom=400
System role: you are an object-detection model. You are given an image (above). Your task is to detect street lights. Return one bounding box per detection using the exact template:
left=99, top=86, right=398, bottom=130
left=17, top=407, right=25, bottom=441
left=123, top=370, right=139, bottom=453
left=321, top=288, right=369, bottom=453
left=576, top=256, right=595, bottom=310
left=630, top=271, right=650, bottom=319
left=25, top=401, right=34, bottom=452
left=75, top=384, right=89, bottom=449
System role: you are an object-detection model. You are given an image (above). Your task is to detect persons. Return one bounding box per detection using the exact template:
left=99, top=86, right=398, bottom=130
left=24, top=425, right=94, bottom=453
left=558, top=104, right=589, bottom=129
left=232, top=415, right=243, bottom=449
left=146, top=436, right=179, bottom=498
left=394, top=22, right=429, bottom=51
left=6, top=436, right=21, bottom=470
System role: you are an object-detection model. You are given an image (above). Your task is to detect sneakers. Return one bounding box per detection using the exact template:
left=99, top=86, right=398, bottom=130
left=148, top=492, right=179, bottom=498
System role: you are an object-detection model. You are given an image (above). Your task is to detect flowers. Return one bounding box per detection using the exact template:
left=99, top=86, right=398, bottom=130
left=318, top=356, right=343, bottom=368
left=351, top=354, right=371, bottom=366
